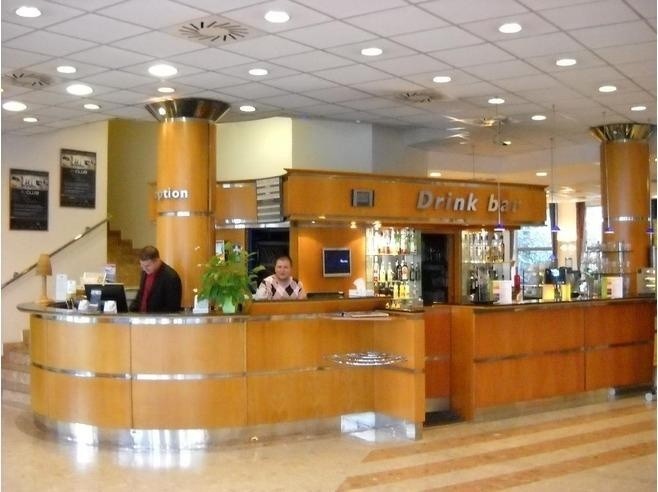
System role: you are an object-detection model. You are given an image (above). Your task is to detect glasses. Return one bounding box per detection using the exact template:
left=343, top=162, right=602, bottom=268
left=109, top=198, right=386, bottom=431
left=603, top=111, right=615, bottom=235
left=493, top=120, right=505, bottom=232
left=34, top=254, right=54, bottom=306
left=551, top=104, right=560, bottom=233
left=646, top=198, right=655, bottom=235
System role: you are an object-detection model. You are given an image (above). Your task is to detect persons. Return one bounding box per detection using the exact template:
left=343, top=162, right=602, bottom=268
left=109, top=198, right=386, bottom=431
left=256, top=256, right=307, bottom=301
left=129, top=247, right=183, bottom=314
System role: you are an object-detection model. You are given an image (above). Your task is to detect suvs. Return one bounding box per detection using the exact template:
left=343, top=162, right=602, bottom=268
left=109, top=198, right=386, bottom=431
left=602, top=277, right=623, bottom=299
left=491, top=280, right=515, bottom=305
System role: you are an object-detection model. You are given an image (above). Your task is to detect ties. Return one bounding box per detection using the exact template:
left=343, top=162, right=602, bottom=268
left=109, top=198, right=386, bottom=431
left=321, top=247, right=352, bottom=280
left=84, top=285, right=129, bottom=314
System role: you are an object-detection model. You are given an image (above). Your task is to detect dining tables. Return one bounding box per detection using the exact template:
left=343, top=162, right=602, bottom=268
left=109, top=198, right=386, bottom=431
left=197, top=241, right=266, bottom=315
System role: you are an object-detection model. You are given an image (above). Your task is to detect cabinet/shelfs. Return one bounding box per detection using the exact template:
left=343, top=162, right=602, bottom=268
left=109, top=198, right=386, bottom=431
left=469, top=271, right=476, bottom=295
left=514, top=267, right=520, bottom=289
left=373, top=256, right=419, bottom=298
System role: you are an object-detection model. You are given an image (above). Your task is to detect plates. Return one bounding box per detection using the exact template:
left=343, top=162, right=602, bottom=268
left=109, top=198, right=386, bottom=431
left=462, top=229, right=510, bottom=296
left=365, top=226, right=422, bottom=300
left=587, top=242, right=633, bottom=281
